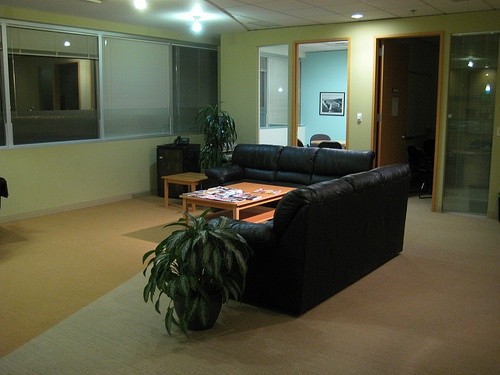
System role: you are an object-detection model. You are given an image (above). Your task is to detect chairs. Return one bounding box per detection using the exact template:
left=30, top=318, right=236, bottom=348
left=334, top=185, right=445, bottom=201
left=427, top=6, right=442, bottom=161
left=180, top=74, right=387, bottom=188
left=407, top=139, right=435, bottom=198
left=310, top=134, right=331, bottom=146
left=318, top=141, right=342, bottom=149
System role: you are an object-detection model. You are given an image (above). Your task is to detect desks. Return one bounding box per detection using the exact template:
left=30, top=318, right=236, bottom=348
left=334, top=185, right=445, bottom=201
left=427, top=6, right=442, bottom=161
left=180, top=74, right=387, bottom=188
left=311, top=140, right=346, bottom=145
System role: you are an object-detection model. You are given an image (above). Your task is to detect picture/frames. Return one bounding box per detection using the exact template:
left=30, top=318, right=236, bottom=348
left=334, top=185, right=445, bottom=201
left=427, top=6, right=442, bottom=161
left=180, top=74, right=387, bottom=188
left=319, top=92, right=345, bottom=116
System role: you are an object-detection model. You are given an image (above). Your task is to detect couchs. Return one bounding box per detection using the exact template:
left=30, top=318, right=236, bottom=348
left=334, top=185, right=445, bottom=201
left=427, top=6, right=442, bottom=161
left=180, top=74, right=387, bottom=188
left=205, top=144, right=376, bottom=209
left=208, top=162, right=412, bottom=318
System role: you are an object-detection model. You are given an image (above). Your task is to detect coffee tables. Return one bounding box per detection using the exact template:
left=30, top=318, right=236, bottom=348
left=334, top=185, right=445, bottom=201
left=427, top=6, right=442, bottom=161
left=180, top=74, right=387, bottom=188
left=178, top=181, right=298, bottom=223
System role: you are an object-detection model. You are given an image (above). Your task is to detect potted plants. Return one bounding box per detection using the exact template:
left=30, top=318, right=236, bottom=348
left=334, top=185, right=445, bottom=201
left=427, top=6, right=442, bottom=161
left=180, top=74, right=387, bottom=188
left=142, top=209, right=253, bottom=332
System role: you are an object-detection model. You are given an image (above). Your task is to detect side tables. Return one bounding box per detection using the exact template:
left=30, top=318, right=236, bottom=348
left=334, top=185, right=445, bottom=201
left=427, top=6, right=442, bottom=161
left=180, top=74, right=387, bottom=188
left=161, top=172, right=208, bottom=212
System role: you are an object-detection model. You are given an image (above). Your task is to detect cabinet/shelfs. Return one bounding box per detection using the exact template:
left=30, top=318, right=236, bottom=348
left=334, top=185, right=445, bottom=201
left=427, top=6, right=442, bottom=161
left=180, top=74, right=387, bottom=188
left=156, top=143, right=200, bottom=199
